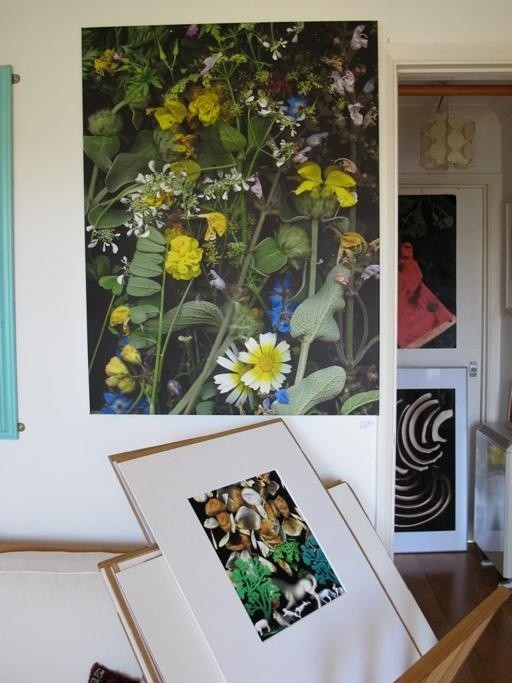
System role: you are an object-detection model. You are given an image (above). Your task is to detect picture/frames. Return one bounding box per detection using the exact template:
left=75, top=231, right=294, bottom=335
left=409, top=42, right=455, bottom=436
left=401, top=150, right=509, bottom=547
left=395, top=367, right=470, bottom=553
